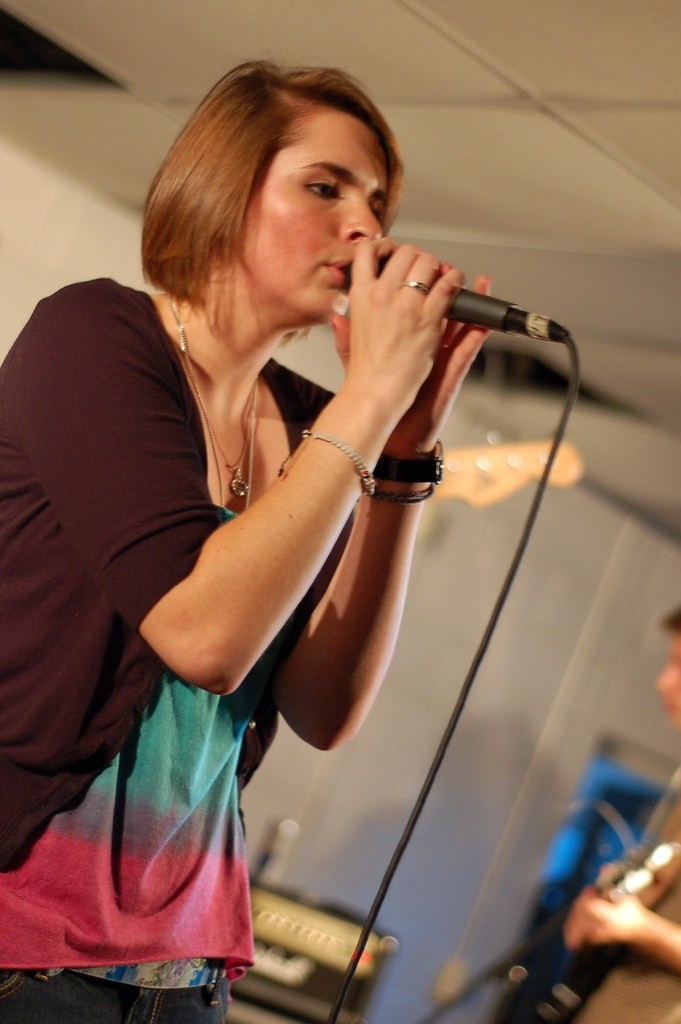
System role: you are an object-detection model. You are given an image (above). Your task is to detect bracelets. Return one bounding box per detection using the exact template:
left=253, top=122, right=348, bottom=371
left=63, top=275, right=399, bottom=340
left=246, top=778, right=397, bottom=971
left=278, top=430, right=376, bottom=496
left=369, top=483, right=435, bottom=504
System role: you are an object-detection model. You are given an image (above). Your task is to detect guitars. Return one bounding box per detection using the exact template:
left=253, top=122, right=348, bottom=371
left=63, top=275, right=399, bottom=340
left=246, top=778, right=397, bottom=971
left=485, top=838, right=681, bottom=1024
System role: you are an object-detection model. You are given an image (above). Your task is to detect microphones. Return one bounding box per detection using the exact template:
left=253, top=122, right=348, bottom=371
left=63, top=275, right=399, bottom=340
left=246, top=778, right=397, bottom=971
left=344, top=254, right=572, bottom=345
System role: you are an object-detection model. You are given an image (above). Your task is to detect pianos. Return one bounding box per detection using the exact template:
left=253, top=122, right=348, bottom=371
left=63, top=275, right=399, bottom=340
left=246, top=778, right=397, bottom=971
left=222, top=873, right=402, bottom=1024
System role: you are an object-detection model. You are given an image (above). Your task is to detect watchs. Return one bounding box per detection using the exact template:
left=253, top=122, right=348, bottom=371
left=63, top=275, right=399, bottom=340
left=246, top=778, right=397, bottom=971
left=372, top=438, right=443, bottom=485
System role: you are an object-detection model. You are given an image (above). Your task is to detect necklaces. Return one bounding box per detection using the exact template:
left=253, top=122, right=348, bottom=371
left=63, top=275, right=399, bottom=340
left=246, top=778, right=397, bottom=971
left=164, top=291, right=258, bottom=512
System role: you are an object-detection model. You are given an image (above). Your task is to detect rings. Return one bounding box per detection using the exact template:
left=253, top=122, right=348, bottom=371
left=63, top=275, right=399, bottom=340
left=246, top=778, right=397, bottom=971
left=401, top=280, right=430, bottom=296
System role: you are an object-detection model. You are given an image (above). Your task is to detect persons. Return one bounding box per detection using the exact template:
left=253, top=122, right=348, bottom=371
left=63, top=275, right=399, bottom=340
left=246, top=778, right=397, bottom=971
left=0, top=59, right=491, bottom=1024
left=563, top=604, right=681, bottom=1024
